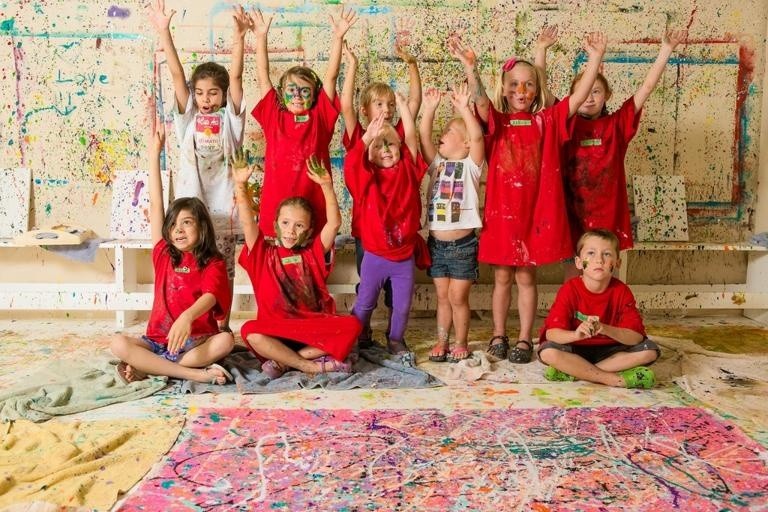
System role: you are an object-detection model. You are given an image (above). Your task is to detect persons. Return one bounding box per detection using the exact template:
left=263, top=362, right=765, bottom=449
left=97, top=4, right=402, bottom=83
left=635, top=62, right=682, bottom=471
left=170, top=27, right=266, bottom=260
left=538, top=228, right=661, bottom=389
left=447, top=30, right=609, bottom=366
left=248, top=4, right=361, bottom=251
left=344, top=91, right=424, bottom=355
left=227, top=145, right=364, bottom=381
left=416, top=81, right=488, bottom=364
left=534, top=24, right=687, bottom=280
left=340, top=39, right=424, bottom=339
left=144, top=1, right=252, bottom=341
left=107, top=118, right=237, bottom=387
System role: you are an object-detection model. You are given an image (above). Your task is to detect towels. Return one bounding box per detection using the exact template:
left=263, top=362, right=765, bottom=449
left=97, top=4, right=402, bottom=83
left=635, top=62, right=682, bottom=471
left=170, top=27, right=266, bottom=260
left=1, top=416, right=187, bottom=510
left=175, top=340, right=448, bottom=398
left=749, top=231, right=768, bottom=249
left=38, top=238, right=116, bottom=263
left=2, top=340, right=170, bottom=421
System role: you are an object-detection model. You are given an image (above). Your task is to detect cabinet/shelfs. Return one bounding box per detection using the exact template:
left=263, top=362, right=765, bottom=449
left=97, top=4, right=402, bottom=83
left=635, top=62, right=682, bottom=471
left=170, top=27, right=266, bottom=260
left=0, top=235, right=768, bottom=325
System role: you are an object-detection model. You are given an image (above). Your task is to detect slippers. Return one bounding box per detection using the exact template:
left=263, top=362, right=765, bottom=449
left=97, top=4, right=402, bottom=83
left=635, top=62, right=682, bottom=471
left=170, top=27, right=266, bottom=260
left=446, top=345, right=472, bottom=363
left=429, top=346, right=450, bottom=361
left=261, top=359, right=289, bottom=379
left=115, top=361, right=148, bottom=385
left=542, top=366, right=575, bottom=382
left=201, top=362, right=234, bottom=385
left=311, top=356, right=352, bottom=374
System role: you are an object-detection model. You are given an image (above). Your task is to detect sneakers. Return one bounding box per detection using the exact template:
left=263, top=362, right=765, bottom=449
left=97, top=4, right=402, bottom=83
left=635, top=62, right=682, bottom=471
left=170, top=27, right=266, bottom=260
left=359, top=336, right=374, bottom=349
left=485, top=335, right=510, bottom=362
left=509, top=338, right=534, bottom=363
left=616, top=366, right=657, bottom=390
left=387, top=339, right=412, bottom=355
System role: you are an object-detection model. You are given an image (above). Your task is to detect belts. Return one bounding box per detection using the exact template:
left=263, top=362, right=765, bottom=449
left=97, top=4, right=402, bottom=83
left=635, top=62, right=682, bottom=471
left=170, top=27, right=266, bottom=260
left=428, top=230, right=476, bottom=248
